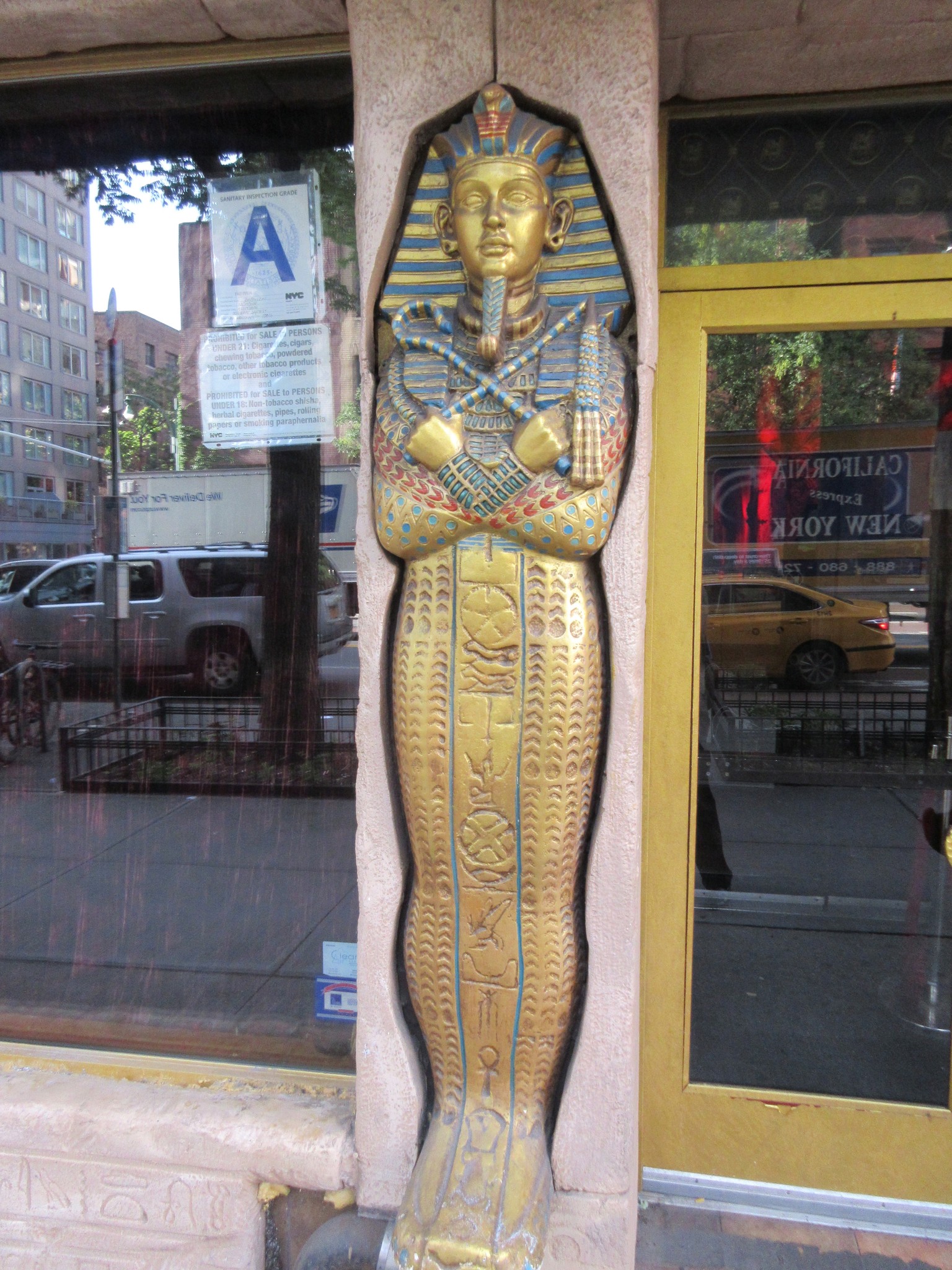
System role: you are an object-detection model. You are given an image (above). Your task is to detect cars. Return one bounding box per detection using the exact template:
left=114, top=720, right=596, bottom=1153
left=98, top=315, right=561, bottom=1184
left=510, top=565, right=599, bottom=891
left=701, top=548, right=896, bottom=691
left=886, top=601, right=930, bottom=666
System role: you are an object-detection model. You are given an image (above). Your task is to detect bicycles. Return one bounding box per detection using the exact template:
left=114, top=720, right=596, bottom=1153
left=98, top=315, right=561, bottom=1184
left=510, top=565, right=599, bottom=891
left=0, top=639, right=64, bottom=765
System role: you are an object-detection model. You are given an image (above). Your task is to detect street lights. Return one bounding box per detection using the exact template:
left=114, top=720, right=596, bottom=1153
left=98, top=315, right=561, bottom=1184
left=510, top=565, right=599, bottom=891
left=125, top=394, right=179, bottom=472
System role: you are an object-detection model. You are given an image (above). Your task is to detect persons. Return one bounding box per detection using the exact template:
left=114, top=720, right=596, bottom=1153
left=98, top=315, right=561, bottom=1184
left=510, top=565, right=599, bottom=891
left=698, top=622, right=737, bottom=893
left=369, top=100, right=631, bottom=1270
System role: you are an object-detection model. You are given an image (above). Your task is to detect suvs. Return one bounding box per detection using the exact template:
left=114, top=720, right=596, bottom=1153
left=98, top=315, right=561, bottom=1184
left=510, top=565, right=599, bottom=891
left=0, top=541, right=359, bottom=701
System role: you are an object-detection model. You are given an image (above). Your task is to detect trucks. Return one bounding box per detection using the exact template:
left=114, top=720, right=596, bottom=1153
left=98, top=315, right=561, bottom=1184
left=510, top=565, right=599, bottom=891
left=107, top=463, right=362, bottom=617
